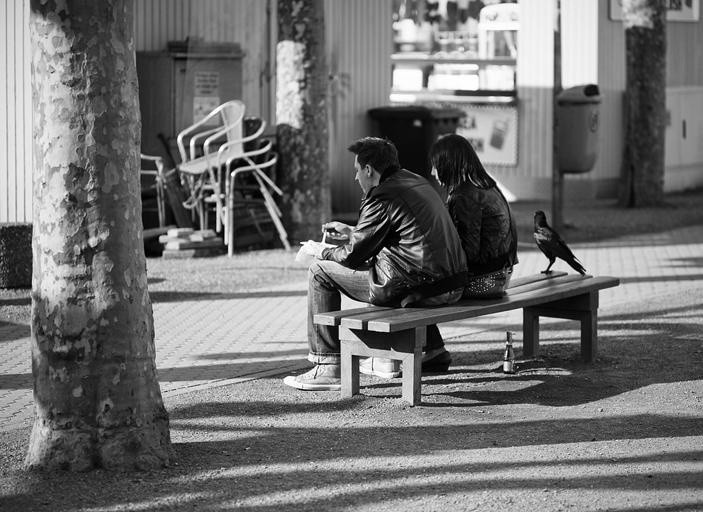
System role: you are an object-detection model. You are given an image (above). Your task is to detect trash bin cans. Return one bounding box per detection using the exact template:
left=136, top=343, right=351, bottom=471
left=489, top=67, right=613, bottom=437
left=369, top=102, right=468, bottom=203
left=556, top=83, right=603, bottom=174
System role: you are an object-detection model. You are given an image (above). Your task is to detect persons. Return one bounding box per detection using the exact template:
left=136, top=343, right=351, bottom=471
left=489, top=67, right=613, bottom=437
left=412, top=133, right=518, bottom=371
left=282, top=135, right=467, bottom=391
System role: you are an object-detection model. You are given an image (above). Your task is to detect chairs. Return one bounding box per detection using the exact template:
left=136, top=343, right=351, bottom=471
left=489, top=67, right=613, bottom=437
left=176, top=99, right=291, bottom=258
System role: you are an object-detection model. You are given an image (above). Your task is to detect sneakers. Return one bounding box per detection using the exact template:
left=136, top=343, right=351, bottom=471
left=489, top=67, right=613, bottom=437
left=359, top=359, right=400, bottom=379
left=285, top=364, right=340, bottom=391
left=421, top=352, right=450, bottom=372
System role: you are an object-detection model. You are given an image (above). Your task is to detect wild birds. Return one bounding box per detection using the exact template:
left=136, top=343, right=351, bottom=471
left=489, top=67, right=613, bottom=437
left=529, top=210, right=587, bottom=276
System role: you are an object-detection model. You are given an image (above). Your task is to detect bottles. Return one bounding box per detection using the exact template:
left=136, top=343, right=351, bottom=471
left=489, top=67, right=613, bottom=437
left=503, top=332, right=515, bottom=374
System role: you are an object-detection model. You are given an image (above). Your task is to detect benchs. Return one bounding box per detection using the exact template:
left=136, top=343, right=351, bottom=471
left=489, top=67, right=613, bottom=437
left=312, top=270, right=620, bottom=407
